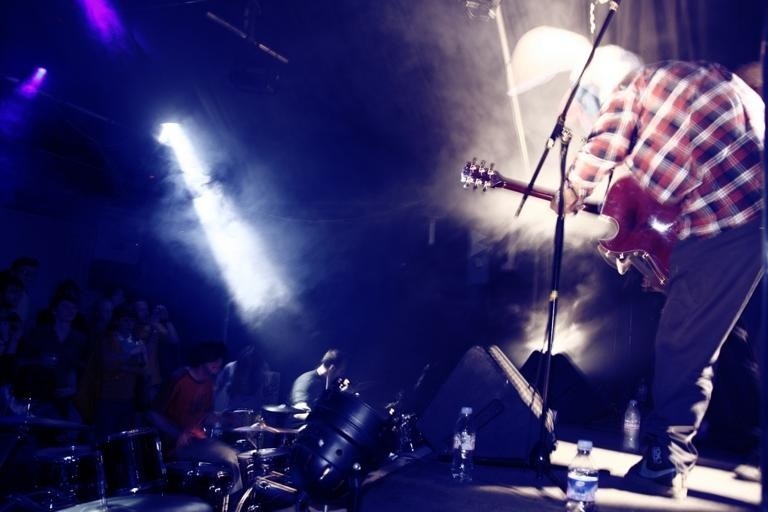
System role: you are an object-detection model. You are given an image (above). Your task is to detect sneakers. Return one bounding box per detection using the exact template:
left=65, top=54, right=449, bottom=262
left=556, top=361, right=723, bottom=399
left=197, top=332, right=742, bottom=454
left=623, top=447, right=688, bottom=499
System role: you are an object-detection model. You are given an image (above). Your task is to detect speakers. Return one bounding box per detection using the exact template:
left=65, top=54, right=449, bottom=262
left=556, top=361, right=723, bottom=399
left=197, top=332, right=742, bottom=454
left=518, top=351, right=609, bottom=424
left=416, top=342, right=555, bottom=467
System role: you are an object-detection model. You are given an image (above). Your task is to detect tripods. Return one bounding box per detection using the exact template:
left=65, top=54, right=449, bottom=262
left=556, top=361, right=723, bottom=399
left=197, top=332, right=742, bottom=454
left=474, top=130, right=611, bottom=512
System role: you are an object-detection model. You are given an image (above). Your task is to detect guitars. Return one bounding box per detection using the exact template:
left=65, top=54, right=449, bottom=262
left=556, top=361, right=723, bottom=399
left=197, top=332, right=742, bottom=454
left=460, top=156, right=683, bottom=292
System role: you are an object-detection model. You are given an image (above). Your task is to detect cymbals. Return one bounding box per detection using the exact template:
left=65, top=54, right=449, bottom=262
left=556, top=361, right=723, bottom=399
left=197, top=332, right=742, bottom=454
left=232, top=422, right=280, bottom=434
left=262, top=404, right=306, bottom=414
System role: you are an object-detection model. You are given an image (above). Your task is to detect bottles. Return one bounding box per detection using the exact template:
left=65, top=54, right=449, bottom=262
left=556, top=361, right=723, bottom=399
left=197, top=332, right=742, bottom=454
left=622, top=398, right=643, bottom=453
left=449, top=405, right=477, bottom=482
left=563, top=439, right=598, bottom=512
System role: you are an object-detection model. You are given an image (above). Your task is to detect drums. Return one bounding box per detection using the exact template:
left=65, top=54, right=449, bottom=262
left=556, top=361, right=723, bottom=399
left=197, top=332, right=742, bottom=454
left=237, top=448, right=291, bottom=488
left=32, top=445, right=105, bottom=511
left=101, top=427, right=169, bottom=498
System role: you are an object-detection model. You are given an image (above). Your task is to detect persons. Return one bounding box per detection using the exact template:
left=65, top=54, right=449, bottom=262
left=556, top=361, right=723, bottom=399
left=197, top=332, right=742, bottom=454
left=0, top=244, right=350, bottom=512
left=730, top=60, right=768, bottom=485
left=549, top=44, right=768, bottom=498
